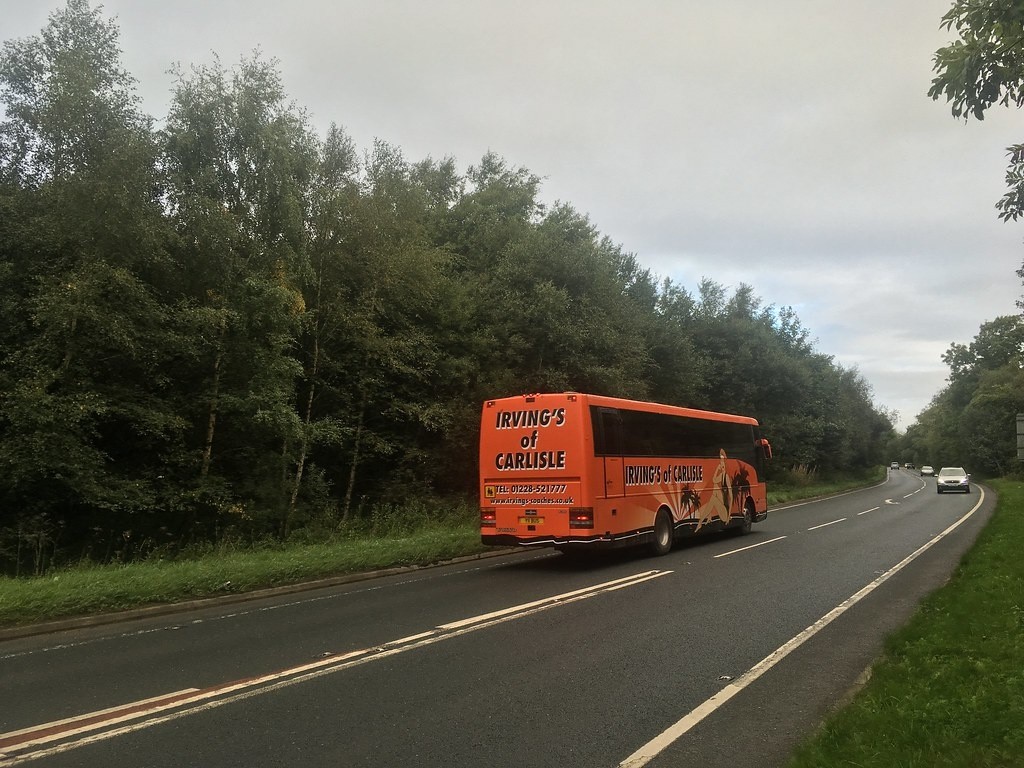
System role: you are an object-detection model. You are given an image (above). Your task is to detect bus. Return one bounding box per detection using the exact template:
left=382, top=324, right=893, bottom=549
left=479, top=391, right=773, bottom=556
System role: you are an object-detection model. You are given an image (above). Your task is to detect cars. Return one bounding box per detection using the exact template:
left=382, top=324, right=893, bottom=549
left=904, top=463, right=909, bottom=468
left=891, top=462, right=900, bottom=470
left=921, top=466, right=935, bottom=477
left=934, top=466, right=972, bottom=494
left=907, top=463, right=914, bottom=469
